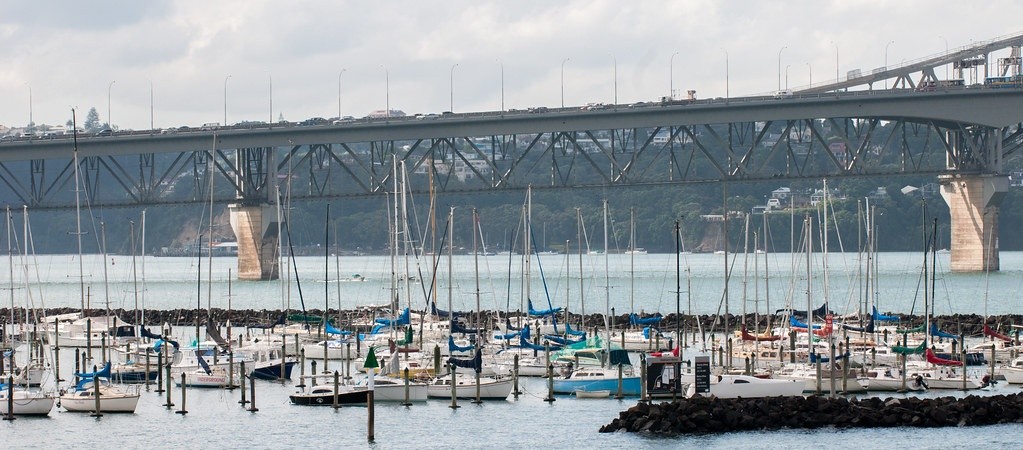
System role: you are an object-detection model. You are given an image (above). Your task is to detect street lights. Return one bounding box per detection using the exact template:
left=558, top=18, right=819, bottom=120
left=611, top=47, right=729, bottom=106
left=786, top=62, right=812, bottom=91
left=338, top=68, right=347, bottom=118
left=561, top=58, right=570, bottom=107
left=450, top=64, right=459, bottom=112
left=778, top=46, right=788, bottom=90
left=105, top=73, right=232, bottom=126
left=884, top=40, right=894, bottom=89
left=830, top=41, right=840, bottom=96
left=496, top=59, right=505, bottom=112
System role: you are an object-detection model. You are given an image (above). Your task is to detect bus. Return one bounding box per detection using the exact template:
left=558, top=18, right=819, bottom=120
left=915, top=74, right=1023, bottom=91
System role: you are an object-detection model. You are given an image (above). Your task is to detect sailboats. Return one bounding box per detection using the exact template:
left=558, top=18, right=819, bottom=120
left=0, top=109, right=1023, bottom=418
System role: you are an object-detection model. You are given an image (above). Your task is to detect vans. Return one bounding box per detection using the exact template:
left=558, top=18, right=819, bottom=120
left=199, top=122, right=221, bottom=131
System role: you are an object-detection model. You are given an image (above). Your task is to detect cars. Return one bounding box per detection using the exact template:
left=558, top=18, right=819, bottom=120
left=528, top=106, right=549, bottom=113
left=233, top=113, right=440, bottom=125
left=774, top=90, right=793, bottom=98
left=2, top=126, right=191, bottom=140
left=580, top=102, right=611, bottom=111
left=628, top=101, right=655, bottom=108
